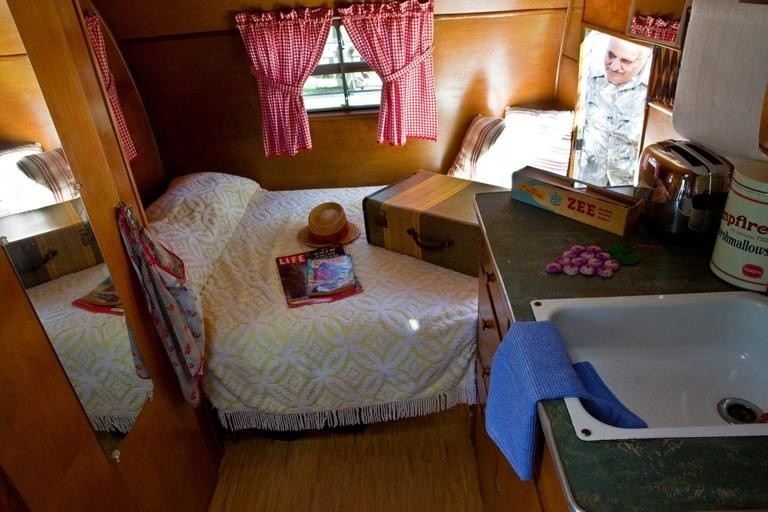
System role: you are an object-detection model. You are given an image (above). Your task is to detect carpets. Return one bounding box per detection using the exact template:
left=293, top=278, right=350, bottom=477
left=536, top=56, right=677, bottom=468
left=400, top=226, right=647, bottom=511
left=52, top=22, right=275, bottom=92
left=94, top=434, right=126, bottom=460
left=207, top=406, right=488, bottom=512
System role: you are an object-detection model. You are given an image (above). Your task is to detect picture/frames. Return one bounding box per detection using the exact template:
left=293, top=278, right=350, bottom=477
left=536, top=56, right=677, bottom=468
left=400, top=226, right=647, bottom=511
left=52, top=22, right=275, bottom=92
left=568, top=21, right=656, bottom=188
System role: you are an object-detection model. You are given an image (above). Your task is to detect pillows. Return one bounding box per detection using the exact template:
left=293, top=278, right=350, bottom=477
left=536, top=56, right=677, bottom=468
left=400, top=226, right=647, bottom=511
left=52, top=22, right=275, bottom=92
left=15, top=148, right=81, bottom=203
left=447, top=113, right=506, bottom=180
left=505, top=104, right=573, bottom=175
left=0, top=142, right=42, bottom=163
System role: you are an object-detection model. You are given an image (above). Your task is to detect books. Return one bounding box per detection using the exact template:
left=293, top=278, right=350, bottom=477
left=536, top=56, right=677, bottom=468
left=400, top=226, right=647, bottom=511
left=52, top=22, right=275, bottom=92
left=305, top=255, right=356, bottom=295
left=276, top=245, right=363, bottom=308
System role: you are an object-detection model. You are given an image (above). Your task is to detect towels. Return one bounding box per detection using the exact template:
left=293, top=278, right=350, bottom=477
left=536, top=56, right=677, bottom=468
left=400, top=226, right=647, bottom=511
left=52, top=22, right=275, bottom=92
left=485, top=322, right=648, bottom=481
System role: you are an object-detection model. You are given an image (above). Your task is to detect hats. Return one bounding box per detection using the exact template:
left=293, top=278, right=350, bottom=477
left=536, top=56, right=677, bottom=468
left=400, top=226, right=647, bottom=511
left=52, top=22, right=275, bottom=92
left=296, top=202, right=361, bottom=248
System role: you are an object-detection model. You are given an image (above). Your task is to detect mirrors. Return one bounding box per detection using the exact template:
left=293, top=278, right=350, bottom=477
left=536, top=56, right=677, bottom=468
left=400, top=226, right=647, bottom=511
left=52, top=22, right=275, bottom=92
left=0, top=0, right=154, bottom=465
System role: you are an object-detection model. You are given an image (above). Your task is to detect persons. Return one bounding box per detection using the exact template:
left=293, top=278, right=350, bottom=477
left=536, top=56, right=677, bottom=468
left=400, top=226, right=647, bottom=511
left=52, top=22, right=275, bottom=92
left=575, top=33, right=650, bottom=185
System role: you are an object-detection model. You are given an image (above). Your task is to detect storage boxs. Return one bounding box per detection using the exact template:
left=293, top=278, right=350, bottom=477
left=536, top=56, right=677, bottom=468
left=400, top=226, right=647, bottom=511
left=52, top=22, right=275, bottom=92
left=511, top=166, right=645, bottom=236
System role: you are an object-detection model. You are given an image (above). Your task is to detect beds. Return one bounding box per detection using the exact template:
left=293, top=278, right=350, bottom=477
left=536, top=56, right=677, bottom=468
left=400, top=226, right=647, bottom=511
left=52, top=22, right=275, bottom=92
left=25, top=261, right=154, bottom=434
left=145, top=172, right=478, bottom=430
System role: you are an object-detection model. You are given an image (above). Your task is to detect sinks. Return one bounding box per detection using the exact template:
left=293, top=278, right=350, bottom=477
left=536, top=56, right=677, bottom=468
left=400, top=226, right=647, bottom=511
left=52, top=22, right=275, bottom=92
left=529, top=292, right=768, bottom=442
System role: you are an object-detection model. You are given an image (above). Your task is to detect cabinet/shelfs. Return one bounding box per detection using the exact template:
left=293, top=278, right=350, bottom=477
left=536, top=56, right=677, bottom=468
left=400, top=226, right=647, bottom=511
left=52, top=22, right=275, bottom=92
left=471, top=237, right=568, bottom=512
left=0, top=0, right=225, bottom=512
